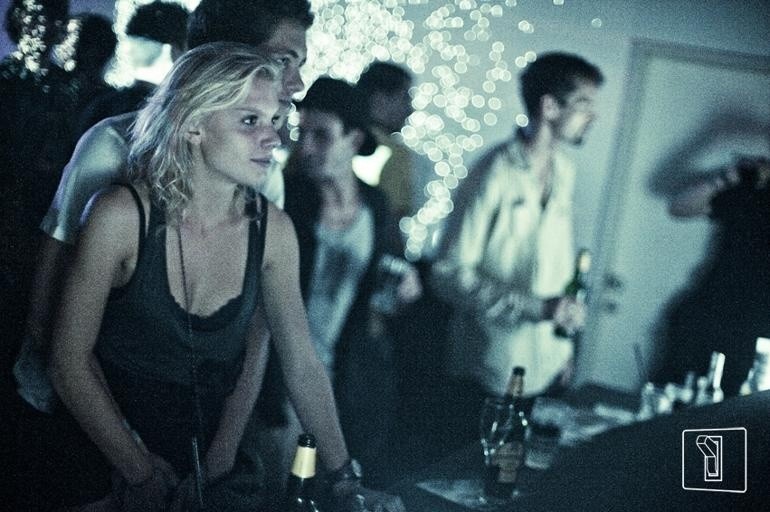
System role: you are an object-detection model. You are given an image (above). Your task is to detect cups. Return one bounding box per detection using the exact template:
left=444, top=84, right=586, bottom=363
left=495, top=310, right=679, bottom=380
left=641, top=381, right=692, bottom=423
left=524, top=397, right=573, bottom=469
left=484, top=396, right=504, bottom=437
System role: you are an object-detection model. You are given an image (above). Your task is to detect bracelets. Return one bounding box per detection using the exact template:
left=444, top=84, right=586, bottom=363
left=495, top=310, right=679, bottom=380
left=712, top=166, right=730, bottom=191
left=129, top=459, right=167, bottom=489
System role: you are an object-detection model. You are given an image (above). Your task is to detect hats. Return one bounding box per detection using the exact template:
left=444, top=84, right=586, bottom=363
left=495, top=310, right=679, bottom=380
left=293, top=77, right=377, bottom=155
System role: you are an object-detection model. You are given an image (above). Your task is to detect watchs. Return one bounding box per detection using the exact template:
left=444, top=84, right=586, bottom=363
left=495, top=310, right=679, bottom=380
left=325, top=459, right=366, bottom=492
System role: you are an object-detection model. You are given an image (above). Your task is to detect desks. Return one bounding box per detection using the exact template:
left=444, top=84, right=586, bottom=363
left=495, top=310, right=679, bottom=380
left=406, top=382, right=642, bottom=510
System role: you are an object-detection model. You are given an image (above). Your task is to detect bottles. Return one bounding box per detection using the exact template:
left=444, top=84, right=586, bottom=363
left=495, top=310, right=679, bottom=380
left=278, top=433, right=318, bottom=511
left=740, top=336, right=770, bottom=399
left=702, top=348, right=725, bottom=404
left=482, top=367, right=532, bottom=499
left=551, top=247, right=592, bottom=339
left=737, top=158, right=768, bottom=187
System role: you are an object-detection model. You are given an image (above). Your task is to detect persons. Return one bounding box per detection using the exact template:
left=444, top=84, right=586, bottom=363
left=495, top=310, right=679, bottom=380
left=649, top=151, right=770, bottom=399
left=46, top=42, right=407, bottom=511
left=64, top=11, right=115, bottom=127
left=223, top=74, right=384, bottom=511
left=72, top=13, right=195, bottom=140
left=1, top=0, right=315, bottom=511
left=425, top=52, right=605, bottom=430
left=351, top=53, right=436, bottom=256
left=0, top=1, right=71, bottom=387
